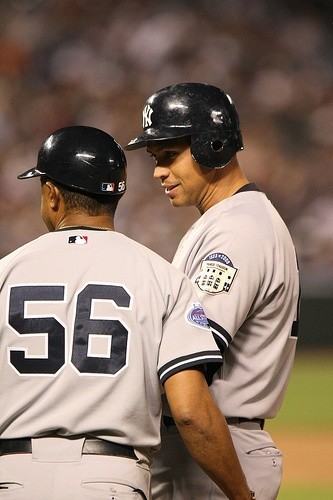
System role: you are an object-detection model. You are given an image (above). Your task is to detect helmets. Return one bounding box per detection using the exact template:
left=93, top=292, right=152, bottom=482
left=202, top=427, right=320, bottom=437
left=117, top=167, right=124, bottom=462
left=124, top=83, right=244, bottom=169
left=17, top=126, right=127, bottom=195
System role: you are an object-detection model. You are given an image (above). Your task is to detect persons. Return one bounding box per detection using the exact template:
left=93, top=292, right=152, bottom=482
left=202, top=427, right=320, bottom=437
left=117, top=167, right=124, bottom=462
left=0, top=125, right=255, bottom=499
left=123, top=82, right=300, bottom=498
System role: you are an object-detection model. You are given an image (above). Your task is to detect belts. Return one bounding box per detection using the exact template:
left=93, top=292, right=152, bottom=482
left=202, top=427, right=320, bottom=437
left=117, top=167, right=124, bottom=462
left=227, top=415, right=265, bottom=431
left=0, top=438, right=137, bottom=459
